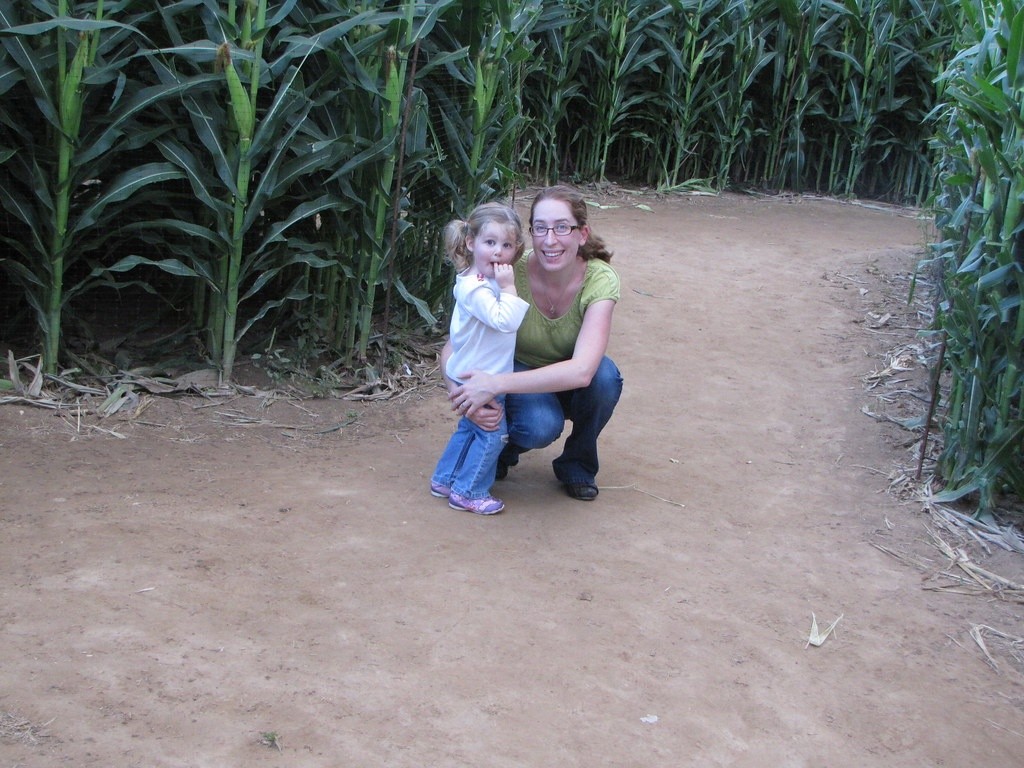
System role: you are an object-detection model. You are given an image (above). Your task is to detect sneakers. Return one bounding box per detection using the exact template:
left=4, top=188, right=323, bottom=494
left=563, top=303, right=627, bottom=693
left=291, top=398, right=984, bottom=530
left=448, top=490, right=505, bottom=515
left=431, top=481, right=450, bottom=497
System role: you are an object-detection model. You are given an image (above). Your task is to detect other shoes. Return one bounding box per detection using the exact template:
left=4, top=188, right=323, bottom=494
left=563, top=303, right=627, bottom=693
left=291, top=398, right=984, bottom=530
left=495, top=461, right=508, bottom=481
left=566, top=482, right=599, bottom=500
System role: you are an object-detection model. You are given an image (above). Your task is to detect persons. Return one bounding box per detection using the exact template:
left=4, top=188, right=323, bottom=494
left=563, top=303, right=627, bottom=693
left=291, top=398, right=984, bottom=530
left=437, top=181, right=622, bottom=502
left=427, top=200, right=532, bottom=517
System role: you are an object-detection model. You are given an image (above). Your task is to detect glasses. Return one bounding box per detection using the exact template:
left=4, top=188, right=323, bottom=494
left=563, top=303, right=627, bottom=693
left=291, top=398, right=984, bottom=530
left=529, top=224, right=581, bottom=237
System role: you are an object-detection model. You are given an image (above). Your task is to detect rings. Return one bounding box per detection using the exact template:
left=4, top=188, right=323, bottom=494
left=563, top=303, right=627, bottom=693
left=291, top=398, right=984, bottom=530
left=460, top=403, right=466, bottom=409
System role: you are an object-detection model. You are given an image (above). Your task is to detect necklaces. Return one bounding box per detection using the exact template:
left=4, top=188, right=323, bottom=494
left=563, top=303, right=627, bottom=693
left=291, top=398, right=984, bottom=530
left=547, top=268, right=575, bottom=314
